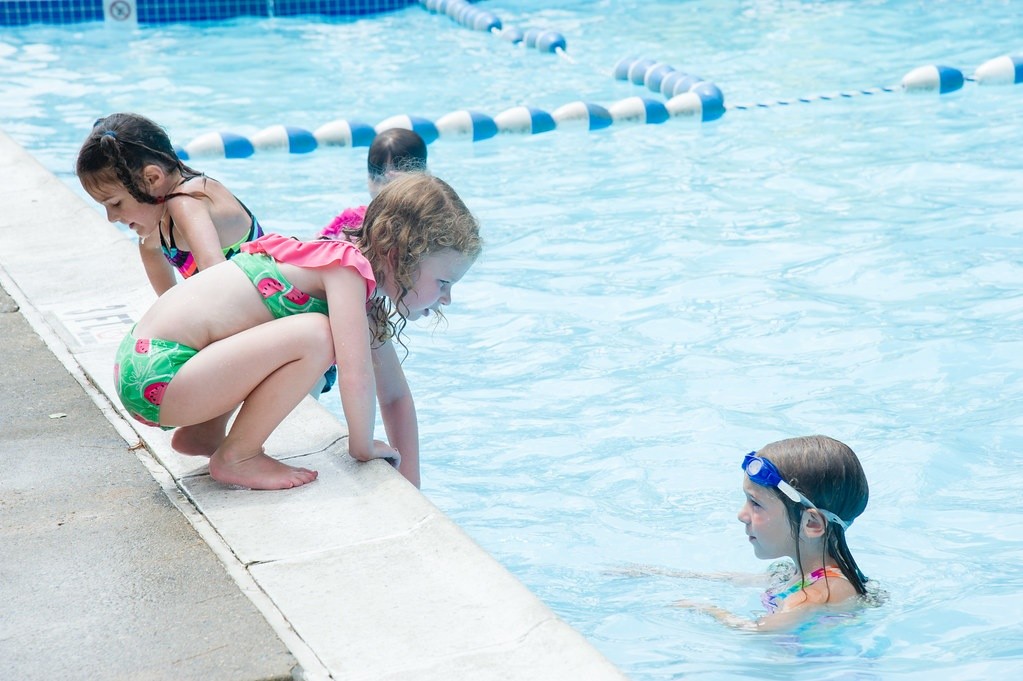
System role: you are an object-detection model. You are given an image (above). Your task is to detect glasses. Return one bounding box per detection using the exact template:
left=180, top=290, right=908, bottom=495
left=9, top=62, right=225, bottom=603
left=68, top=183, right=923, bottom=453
left=741, top=450, right=802, bottom=503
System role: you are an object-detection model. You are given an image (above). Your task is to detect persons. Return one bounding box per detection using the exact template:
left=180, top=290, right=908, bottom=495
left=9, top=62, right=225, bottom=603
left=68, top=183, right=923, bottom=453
left=76, top=113, right=264, bottom=297
left=738, top=435, right=869, bottom=607
left=368, top=128, right=427, bottom=198
left=113, top=174, right=482, bottom=492
